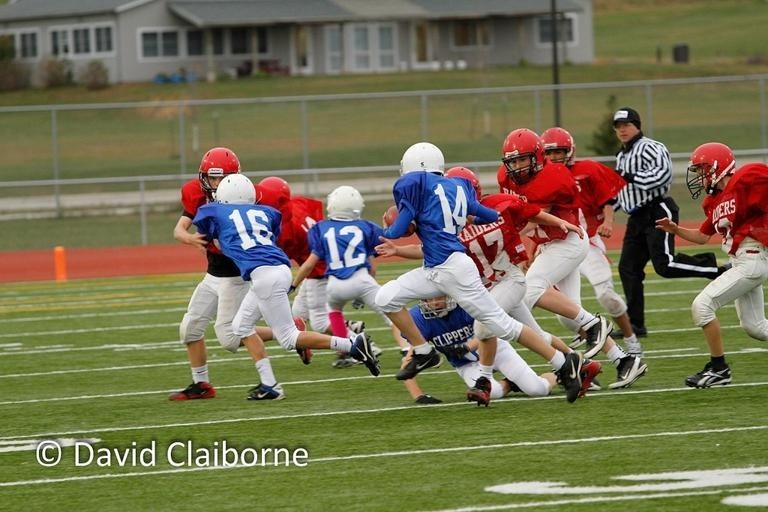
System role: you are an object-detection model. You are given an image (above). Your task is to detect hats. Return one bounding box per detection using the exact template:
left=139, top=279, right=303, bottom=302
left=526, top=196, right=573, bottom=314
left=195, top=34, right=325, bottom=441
left=613, top=108, right=641, bottom=126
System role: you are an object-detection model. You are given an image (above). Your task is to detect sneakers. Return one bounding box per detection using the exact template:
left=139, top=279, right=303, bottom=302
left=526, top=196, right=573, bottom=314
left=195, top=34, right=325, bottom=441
left=248, top=383, right=284, bottom=398
left=554, top=311, right=649, bottom=404
left=395, top=348, right=444, bottom=380
left=332, top=320, right=382, bottom=376
left=294, top=319, right=313, bottom=367
left=686, top=363, right=732, bottom=389
left=466, top=377, right=491, bottom=407
left=170, top=379, right=216, bottom=402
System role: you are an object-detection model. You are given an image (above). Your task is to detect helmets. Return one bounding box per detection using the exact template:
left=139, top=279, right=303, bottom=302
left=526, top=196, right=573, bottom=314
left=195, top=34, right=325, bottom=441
left=326, top=185, right=366, bottom=220
left=199, top=147, right=292, bottom=205
left=686, top=143, right=738, bottom=198
left=398, top=143, right=483, bottom=202
left=501, top=126, right=576, bottom=183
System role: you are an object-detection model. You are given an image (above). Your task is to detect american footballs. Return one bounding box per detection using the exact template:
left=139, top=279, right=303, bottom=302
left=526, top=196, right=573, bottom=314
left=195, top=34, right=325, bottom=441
left=384, top=207, right=416, bottom=237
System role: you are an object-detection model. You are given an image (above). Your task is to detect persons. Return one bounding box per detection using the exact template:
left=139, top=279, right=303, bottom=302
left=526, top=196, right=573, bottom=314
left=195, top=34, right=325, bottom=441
left=655, top=142, right=767, bottom=386
left=609, top=107, right=732, bottom=339
left=169, top=126, right=647, bottom=409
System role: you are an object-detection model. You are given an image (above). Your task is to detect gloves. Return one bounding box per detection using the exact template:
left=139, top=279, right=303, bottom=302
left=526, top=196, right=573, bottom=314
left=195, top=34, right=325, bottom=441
left=415, top=393, right=442, bottom=405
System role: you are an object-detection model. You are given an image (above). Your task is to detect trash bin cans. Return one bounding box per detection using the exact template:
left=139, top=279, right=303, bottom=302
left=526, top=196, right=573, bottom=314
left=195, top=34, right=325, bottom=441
left=673, top=46, right=689, bottom=63
